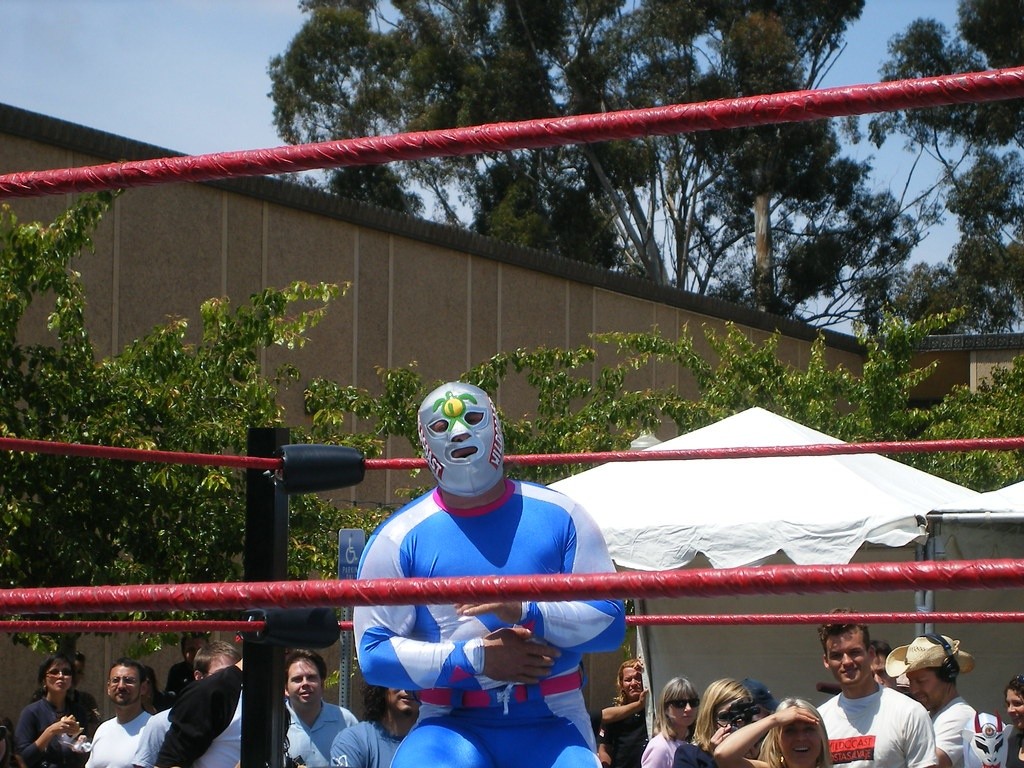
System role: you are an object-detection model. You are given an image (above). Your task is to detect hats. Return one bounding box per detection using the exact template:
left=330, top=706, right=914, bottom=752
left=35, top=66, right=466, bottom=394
left=884, top=634, right=975, bottom=677
left=741, top=678, right=781, bottom=713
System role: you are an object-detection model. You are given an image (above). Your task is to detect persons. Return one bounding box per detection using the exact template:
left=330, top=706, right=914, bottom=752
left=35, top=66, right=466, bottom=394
left=165, top=631, right=210, bottom=706
left=12, top=647, right=101, bottom=768
left=1003, top=673, right=1024, bottom=768
left=639, top=675, right=835, bottom=768
left=814, top=606, right=977, bottom=768
left=585, top=658, right=649, bottom=768
left=0, top=715, right=27, bottom=768
left=328, top=672, right=420, bottom=768
left=84, top=657, right=164, bottom=768
left=131, top=641, right=307, bottom=768
left=352, top=382, right=627, bottom=768
left=234, top=649, right=358, bottom=768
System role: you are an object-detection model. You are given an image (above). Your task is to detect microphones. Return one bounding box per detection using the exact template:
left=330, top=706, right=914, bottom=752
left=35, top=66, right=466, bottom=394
left=815, top=681, right=843, bottom=695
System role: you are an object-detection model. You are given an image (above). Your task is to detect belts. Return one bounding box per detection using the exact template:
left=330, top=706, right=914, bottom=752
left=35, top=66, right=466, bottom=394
left=418, top=671, right=581, bottom=706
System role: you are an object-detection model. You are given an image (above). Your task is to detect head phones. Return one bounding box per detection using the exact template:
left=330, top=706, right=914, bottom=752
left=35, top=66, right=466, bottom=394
left=921, top=633, right=961, bottom=684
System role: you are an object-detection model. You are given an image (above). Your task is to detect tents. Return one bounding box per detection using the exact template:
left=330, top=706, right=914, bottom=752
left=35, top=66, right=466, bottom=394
left=544, top=404, right=1024, bottom=741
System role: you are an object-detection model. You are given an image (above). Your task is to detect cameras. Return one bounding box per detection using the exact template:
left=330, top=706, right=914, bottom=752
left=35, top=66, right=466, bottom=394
left=717, top=695, right=760, bottom=734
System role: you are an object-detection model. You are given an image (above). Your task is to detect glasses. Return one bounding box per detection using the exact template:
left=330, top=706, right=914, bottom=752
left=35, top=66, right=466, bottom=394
left=45, top=668, right=74, bottom=677
left=0, top=726, right=7, bottom=741
left=664, top=698, right=700, bottom=709
left=106, top=676, right=139, bottom=687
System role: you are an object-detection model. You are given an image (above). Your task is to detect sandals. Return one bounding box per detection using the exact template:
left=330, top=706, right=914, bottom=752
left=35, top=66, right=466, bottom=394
left=57, top=728, right=92, bottom=754
